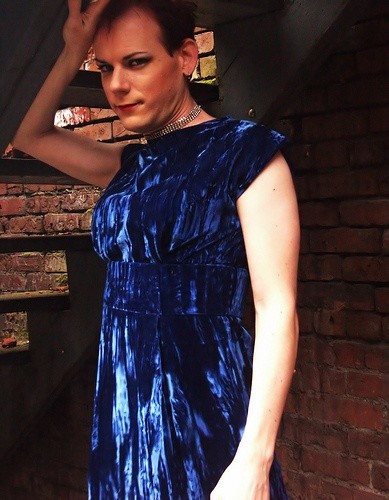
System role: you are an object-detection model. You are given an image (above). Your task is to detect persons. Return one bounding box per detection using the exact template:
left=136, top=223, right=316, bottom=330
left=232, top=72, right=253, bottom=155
left=13, top=0, right=303, bottom=500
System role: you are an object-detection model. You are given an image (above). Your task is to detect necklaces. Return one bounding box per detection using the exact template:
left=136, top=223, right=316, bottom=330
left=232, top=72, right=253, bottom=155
left=146, top=105, right=202, bottom=142
